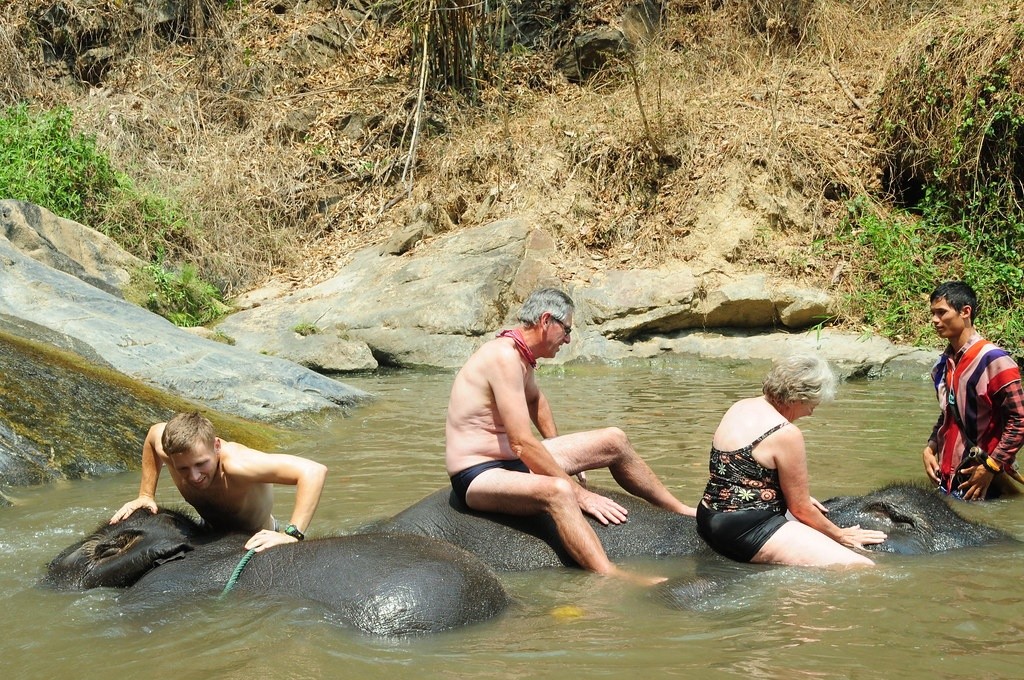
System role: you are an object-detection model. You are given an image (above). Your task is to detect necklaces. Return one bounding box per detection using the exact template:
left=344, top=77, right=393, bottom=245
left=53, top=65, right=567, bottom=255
left=495, top=328, right=537, bottom=368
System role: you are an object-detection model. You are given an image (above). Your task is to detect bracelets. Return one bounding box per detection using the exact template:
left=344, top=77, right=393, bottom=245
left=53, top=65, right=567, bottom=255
left=983, top=455, right=1004, bottom=474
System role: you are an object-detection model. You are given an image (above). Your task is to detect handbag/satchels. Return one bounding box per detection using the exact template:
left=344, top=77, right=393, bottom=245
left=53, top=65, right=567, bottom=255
left=955, top=446, right=1001, bottom=501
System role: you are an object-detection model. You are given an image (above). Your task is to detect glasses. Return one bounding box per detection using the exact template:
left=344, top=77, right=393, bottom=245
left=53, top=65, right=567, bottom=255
left=551, top=316, right=572, bottom=338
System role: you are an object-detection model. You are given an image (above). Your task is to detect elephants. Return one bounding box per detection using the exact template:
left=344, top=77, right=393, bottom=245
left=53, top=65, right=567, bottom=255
left=41, top=472, right=1021, bottom=642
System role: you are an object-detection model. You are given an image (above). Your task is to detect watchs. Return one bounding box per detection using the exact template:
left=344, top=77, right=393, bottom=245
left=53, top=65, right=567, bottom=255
left=283, top=525, right=304, bottom=541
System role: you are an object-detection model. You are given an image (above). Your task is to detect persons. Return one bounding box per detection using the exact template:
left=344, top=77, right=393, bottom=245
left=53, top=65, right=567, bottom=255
left=445, top=288, right=697, bottom=588
left=111, top=410, right=328, bottom=553
left=922, top=281, right=1024, bottom=502
left=696, top=352, right=887, bottom=567
